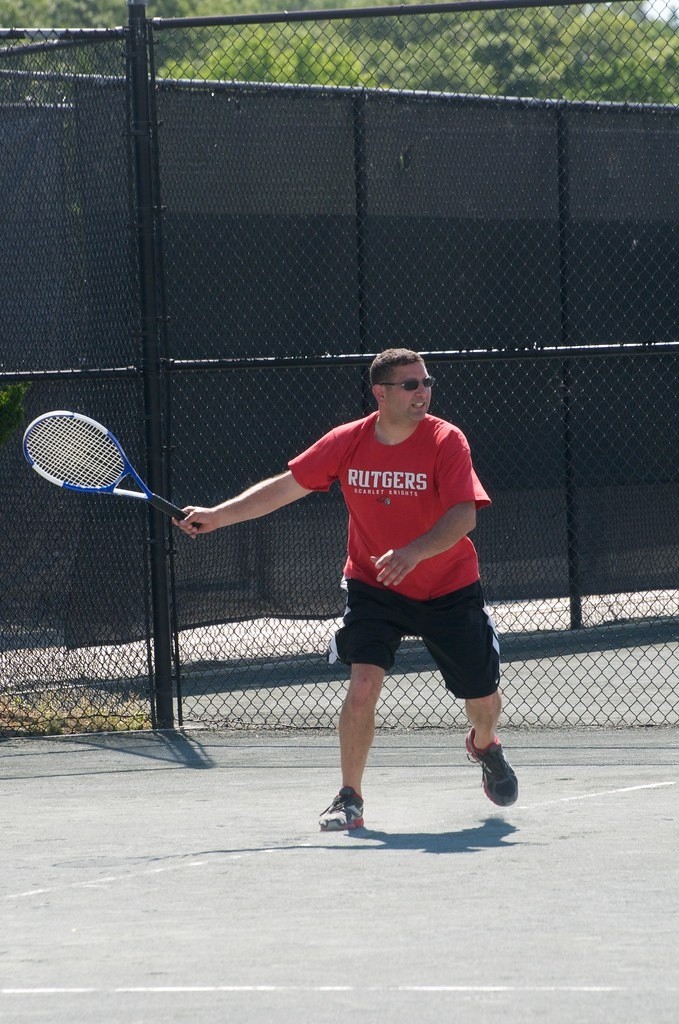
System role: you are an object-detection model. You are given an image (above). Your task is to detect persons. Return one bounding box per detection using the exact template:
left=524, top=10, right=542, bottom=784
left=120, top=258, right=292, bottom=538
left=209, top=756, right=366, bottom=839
left=171, top=348, right=519, bottom=832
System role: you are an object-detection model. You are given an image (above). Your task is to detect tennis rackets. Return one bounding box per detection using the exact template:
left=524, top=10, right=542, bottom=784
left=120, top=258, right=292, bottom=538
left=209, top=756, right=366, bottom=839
left=20, top=409, right=210, bottom=535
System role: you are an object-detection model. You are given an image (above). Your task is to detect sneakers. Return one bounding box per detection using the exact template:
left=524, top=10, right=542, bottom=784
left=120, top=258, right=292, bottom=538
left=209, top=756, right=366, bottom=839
left=318, top=786, right=364, bottom=831
left=465, top=726, right=518, bottom=807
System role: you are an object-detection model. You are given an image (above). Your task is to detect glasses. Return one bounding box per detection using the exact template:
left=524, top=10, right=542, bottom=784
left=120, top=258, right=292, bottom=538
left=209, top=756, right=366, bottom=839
left=380, top=377, right=434, bottom=391
